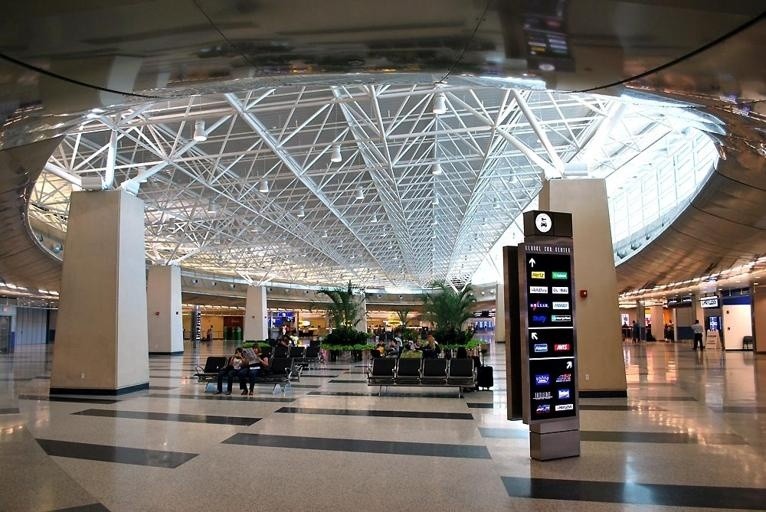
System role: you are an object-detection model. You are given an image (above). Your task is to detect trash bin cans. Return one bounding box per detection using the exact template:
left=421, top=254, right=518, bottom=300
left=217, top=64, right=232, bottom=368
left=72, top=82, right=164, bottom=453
left=225, top=326, right=241, bottom=339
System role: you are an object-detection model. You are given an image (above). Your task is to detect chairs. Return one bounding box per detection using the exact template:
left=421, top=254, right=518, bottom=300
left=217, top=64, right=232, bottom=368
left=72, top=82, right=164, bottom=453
left=193, top=341, right=481, bottom=398
left=743, top=336, right=754, bottom=351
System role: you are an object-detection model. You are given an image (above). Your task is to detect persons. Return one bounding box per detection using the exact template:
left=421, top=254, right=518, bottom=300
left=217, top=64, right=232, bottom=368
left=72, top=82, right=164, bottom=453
left=207, top=324, right=299, bottom=395
left=668, top=319, right=674, bottom=339
left=631, top=320, right=641, bottom=343
left=690, top=319, right=704, bottom=352
left=373, top=333, right=478, bottom=359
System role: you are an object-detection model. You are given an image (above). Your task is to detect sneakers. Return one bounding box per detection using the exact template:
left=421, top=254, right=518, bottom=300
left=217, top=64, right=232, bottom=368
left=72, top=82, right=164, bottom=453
left=216, top=391, right=222, bottom=394
left=250, top=391, right=252, bottom=395
left=241, top=390, right=248, bottom=395
left=226, top=391, right=231, bottom=394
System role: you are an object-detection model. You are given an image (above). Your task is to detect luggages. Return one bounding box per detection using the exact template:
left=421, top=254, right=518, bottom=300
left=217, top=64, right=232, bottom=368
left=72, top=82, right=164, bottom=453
left=476, top=366, right=493, bottom=391
